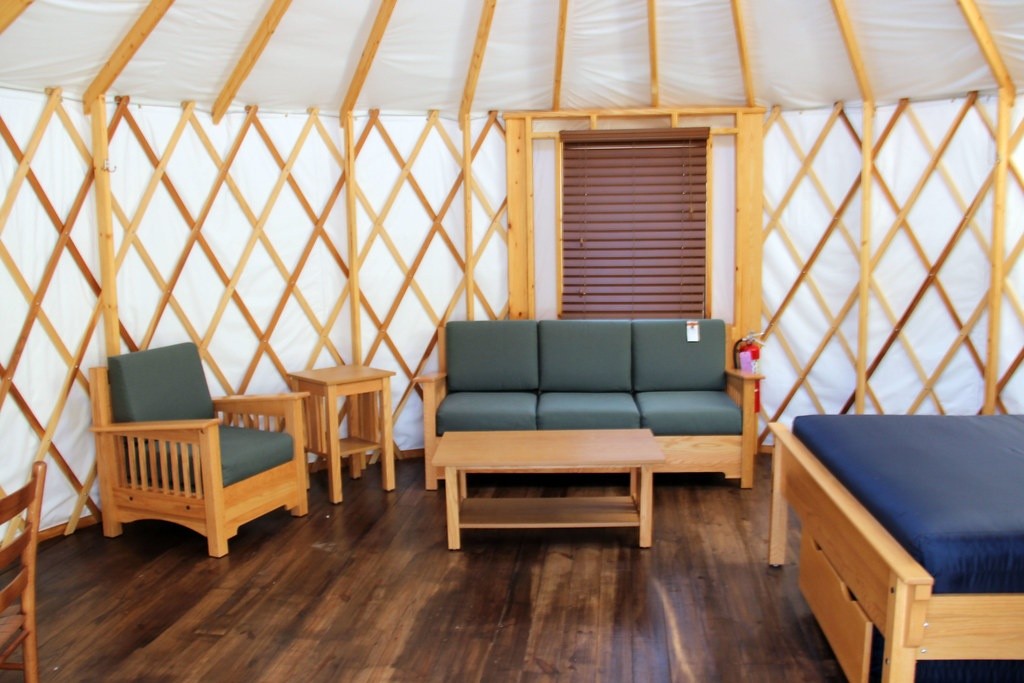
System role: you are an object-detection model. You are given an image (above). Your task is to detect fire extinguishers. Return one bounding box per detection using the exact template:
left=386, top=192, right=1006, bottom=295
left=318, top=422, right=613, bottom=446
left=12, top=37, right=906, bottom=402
left=733, top=333, right=767, bottom=414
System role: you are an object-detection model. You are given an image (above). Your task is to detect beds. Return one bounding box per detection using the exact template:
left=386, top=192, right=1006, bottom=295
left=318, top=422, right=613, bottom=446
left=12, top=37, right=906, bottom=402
left=765, top=413, right=1024, bottom=683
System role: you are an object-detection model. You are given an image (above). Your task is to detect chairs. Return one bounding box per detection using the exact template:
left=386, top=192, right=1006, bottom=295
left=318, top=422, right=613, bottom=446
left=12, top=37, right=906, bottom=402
left=0, top=461, right=47, bottom=683
left=90, top=342, right=312, bottom=558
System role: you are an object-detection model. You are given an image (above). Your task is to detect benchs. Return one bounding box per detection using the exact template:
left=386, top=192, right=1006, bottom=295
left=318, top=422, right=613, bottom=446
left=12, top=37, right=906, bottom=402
left=410, top=318, right=766, bottom=488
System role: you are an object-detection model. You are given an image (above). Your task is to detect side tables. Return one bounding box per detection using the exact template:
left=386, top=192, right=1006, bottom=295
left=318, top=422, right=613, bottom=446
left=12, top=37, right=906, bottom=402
left=285, top=364, right=399, bottom=503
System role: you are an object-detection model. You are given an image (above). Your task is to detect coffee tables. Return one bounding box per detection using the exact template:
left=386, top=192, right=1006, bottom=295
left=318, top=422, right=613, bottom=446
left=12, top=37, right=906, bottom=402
left=430, top=429, right=665, bottom=550
